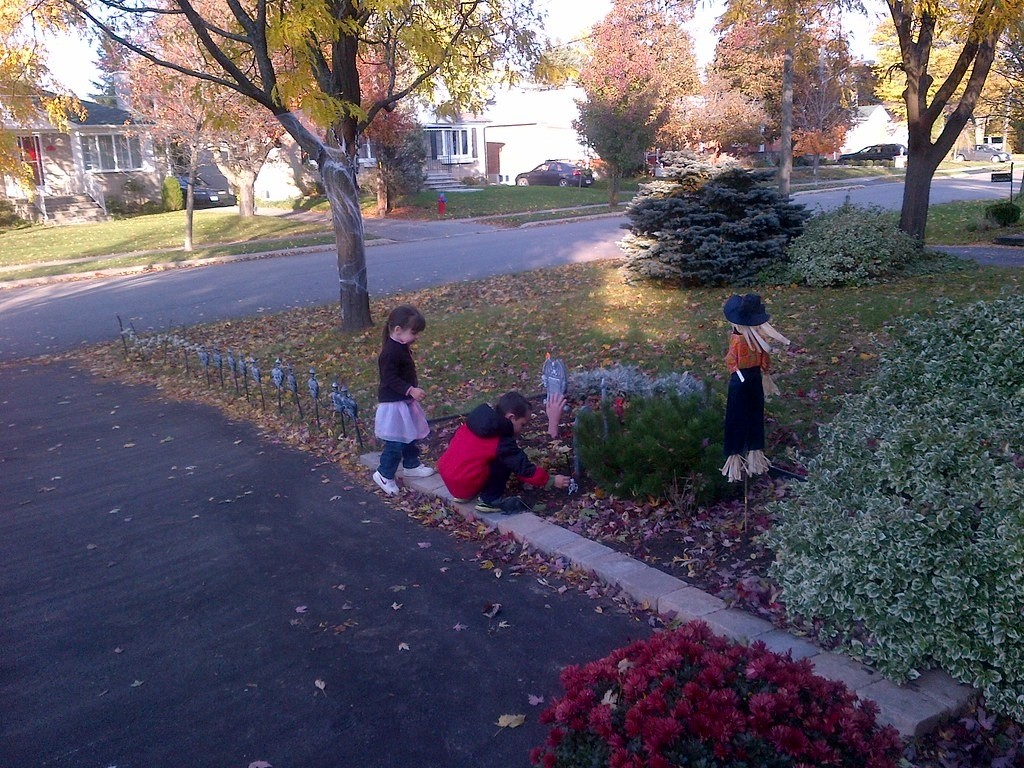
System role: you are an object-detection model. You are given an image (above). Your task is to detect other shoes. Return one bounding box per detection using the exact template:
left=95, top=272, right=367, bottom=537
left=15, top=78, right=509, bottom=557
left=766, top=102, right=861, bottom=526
left=475, top=499, right=501, bottom=512
left=454, top=497, right=470, bottom=503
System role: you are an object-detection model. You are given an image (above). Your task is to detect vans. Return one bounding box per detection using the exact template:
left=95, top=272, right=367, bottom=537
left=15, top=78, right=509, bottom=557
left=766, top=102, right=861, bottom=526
left=982, top=135, right=1004, bottom=150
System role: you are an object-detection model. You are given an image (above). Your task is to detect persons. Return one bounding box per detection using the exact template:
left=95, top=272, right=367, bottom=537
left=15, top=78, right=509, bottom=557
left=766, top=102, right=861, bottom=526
left=372, top=304, right=435, bottom=497
left=719, top=294, right=791, bottom=482
left=436, top=391, right=572, bottom=514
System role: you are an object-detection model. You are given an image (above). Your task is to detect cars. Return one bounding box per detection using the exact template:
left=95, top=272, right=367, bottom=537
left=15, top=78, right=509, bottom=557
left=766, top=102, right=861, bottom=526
left=952, top=144, right=1011, bottom=163
left=515, top=159, right=595, bottom=188
left=837, top=143, right=908, bottom=163
left=175, top=172, right=229, bottom=207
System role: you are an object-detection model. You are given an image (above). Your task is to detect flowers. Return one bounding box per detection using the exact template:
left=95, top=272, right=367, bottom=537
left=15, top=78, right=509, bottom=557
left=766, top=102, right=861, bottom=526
left=528, top=618, right=908, bottom=768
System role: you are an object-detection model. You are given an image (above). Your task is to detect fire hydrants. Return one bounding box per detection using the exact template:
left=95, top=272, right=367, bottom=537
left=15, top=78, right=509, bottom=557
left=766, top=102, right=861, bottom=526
left=437, top=192, right=448, bottom=214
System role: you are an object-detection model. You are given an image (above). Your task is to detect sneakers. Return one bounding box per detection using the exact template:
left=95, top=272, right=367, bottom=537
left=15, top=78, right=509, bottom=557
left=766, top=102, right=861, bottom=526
left=373, top=471, right=399, bottom=497
left=404, top=464, right=434, bottom=477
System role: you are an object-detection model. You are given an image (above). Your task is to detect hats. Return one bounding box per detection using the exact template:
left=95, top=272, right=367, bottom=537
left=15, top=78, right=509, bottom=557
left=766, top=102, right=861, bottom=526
left=723, top=294, right=771, bottom=326
